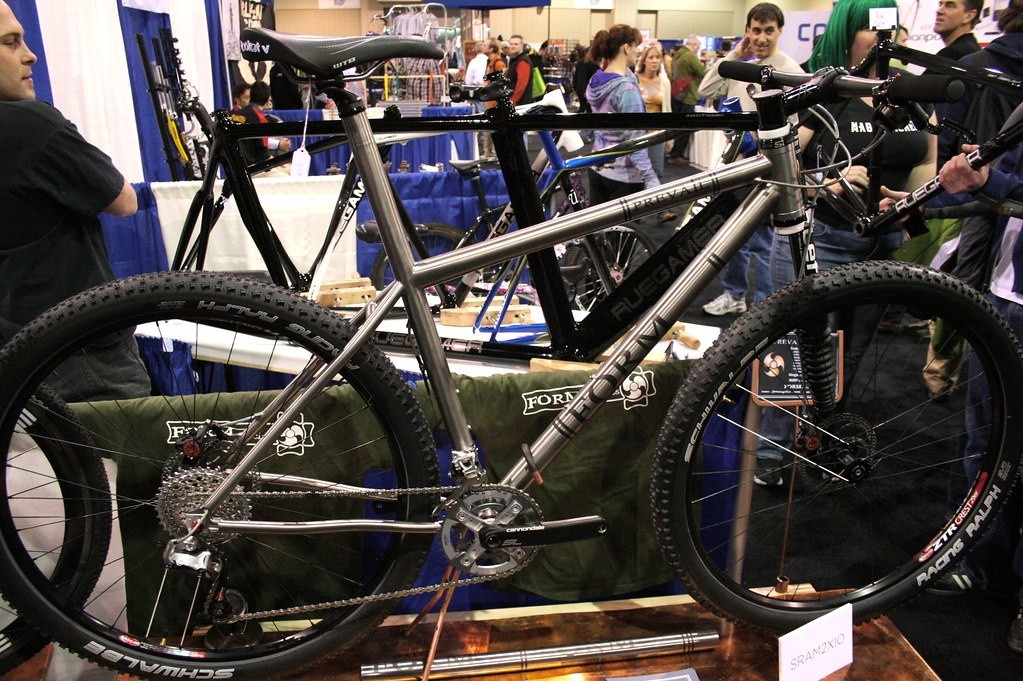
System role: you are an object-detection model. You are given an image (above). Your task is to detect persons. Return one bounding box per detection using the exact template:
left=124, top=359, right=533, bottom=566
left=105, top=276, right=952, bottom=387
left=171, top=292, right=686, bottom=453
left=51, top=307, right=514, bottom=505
left=752, top=0, right=1023, bottom=658
left=585, top=25, right=662, bottom=288
left=395, top=33, right=443, bottom=106
left=661, top=35, right=731, bottom=163
left=697, top=1, right=802, bottom=318
left=460, top=32, right=546, bottom=164
left=228, top=81, right=292, bottom=175
left=630, top=34, right=678, bottom=225
left=270, top=61, right=366, bottom=110
left=0, top=0, right=153, bottom=398
left=564, top=29, right=610, bottom=140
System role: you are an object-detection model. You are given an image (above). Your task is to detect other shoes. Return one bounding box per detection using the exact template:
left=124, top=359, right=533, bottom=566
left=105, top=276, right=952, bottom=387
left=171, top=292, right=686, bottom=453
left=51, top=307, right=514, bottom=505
left=657, top=209, right=677, bottom=225
left=668, top=156, right=691, bottom=164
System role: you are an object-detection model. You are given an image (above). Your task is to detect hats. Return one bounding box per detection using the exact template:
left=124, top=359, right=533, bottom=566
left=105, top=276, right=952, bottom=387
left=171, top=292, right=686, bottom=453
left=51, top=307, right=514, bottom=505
left=500, top=40, right=511, bottom=49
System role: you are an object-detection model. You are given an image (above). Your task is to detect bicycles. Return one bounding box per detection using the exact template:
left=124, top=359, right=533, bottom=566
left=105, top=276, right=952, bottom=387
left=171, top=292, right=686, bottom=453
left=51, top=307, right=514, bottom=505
left=1, top=27, right=1023, bottom=681
left=171, top=29, right=1023, bottom=361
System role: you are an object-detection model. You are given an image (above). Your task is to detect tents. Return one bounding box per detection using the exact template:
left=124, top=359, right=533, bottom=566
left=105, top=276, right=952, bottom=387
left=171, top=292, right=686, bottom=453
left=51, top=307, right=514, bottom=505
left=422, top=0, right=551, bottom=53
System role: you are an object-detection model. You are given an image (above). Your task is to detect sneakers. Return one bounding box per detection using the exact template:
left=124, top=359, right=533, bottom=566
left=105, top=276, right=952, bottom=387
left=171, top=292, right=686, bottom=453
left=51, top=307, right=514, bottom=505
left=703, top=292, right=747, bottom=316
left=923, top=556, right=1023, bottom=654
left=753, top=459, right=785, bottom=486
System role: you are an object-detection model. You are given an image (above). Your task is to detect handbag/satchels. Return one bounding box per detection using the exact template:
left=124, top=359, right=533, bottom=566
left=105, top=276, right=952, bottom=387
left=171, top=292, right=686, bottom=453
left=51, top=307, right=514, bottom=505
left=670, top=74, right=693, bottom=102
left=533, top=66, right=546, bottom=99
